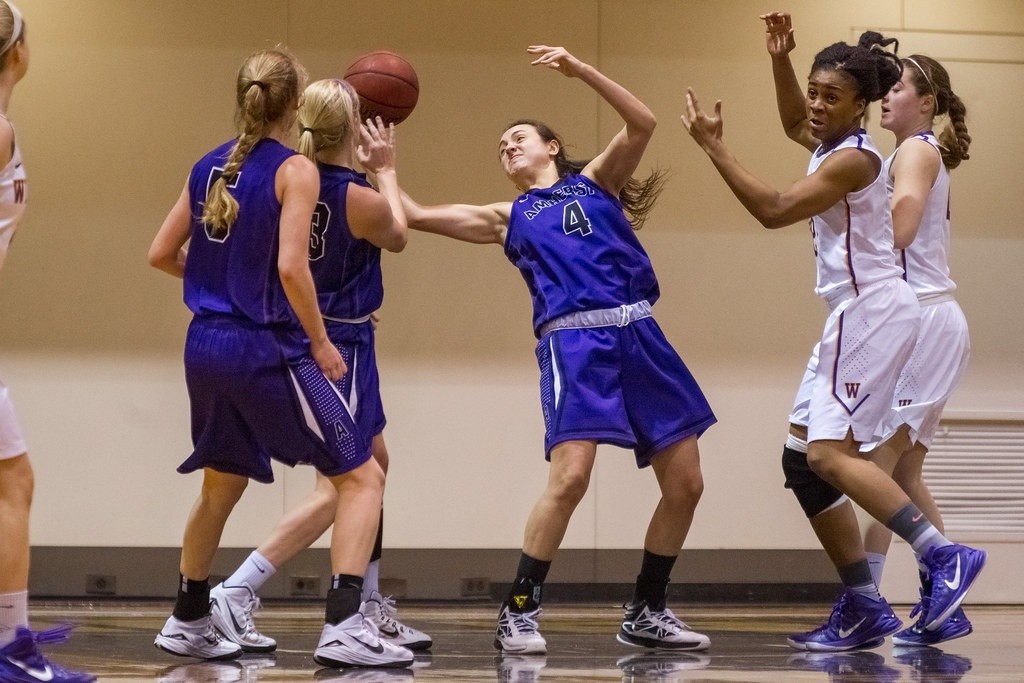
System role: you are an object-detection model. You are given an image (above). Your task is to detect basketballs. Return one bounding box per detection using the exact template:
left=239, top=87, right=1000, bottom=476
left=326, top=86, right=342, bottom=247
left=344, top=49, right=421, bottom=129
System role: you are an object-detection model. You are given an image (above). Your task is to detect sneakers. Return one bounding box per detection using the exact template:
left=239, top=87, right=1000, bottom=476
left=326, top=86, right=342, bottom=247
left=208, top=581, right=277, bottom=653
left=616, top=600, right=711, bottom=651
left=0, top=623, right=97, bottom=683
left=359, top=595, right=433, bottom=652
left=892, top=586, right=973, bottom=645
left=805, top=588, right=903, bottom=652
left=494, top=598, right=547, bottom=654
left=786, top=623, right=885, bottom=650
left=921, top=544, right=986, bottom=631
left=154, top=598, right=243, bottom=659
left=314, top=602, right=414, bottom=668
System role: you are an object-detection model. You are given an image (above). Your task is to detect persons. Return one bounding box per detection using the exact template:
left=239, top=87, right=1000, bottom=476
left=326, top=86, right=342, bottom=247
left=209, top=76, right=433, bottom=648
left=683, top=11, right=987, bottom=653
left=365, top=42, right=717, bottom=654
left=148, top=43, right=413, bottom=666
left=0, top=0, right=98, bottom=683
